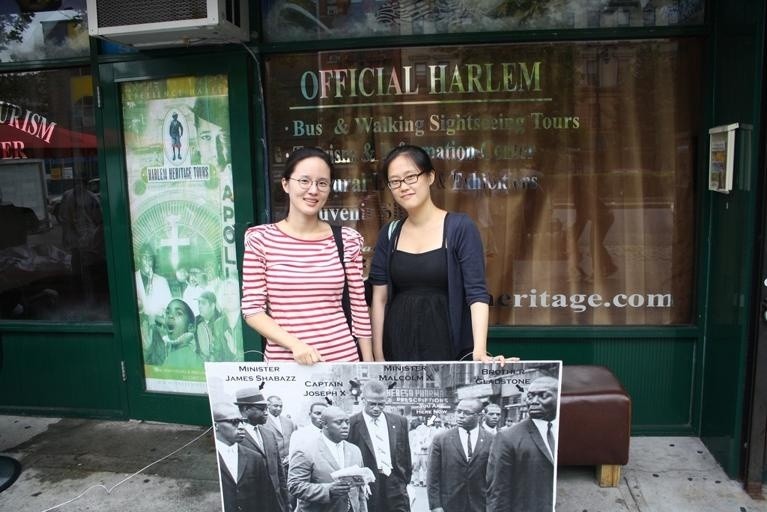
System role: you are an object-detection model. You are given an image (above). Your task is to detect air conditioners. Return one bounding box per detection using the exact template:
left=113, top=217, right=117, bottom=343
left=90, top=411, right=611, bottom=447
left=84, top=1, right=251, bottom=51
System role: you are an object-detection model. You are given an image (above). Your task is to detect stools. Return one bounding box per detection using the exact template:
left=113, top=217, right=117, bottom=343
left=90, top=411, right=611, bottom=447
left=553, top=362, right=629, bottom=489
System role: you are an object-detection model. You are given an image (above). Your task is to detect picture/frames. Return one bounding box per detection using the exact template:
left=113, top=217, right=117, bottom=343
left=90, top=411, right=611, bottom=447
left=707, top=122, right=740, bottom=195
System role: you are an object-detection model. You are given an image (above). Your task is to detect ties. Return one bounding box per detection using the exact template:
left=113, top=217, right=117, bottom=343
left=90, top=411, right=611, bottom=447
left=547, top=422, right=555, bottom=460
left=255, top=427, right=263, bottom=448
left=467, top=432, right=472, bottom=459
left=375, top=419, right=393, bottom=478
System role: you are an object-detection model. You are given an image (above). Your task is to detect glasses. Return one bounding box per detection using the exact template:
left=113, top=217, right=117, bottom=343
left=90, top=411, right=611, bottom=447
left=290, top=177, right=332, bottom=193
left=387, top=172, right=426, bottom=191
left=216, top=418, right=248, bottom=428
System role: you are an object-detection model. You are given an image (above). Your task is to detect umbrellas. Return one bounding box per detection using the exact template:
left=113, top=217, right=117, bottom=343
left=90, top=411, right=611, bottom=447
left=0, top=118, right=99, bottom=158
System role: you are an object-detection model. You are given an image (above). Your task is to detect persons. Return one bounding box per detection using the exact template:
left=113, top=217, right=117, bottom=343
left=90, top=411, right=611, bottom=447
left=134, top=245, right=243, bottom=369
left=367, top=146, right=520, bottom=368
left=52, top=176, right=103, bottom=305
left=192, top=96, right=231, bottom=173
left=240, top=146, right=374, bottom=366
left=562, top=176, right=618, bottom=280
left=433, top=155, right=543, bottom=297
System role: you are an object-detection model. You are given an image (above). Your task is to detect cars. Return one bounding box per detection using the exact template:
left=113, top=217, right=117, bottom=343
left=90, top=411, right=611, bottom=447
left=47, top=175, right=102, bottom=224
left=357, top=165, right=565, bottom=264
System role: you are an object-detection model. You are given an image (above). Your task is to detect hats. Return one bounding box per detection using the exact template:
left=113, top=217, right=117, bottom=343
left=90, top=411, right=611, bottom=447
left=235, top=387, right=270, bottom=405
